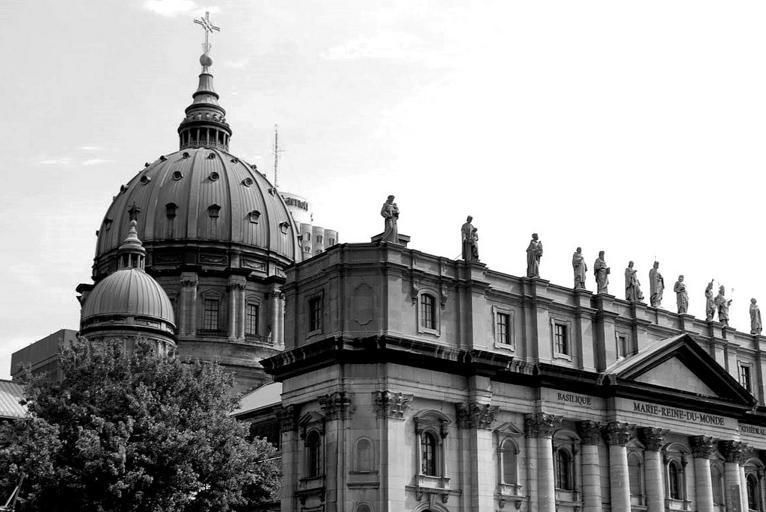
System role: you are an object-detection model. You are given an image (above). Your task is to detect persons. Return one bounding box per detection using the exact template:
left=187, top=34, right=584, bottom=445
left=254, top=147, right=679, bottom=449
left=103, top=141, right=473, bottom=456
left=673, top=274, right=691, bottom=314
left=748, top=297, right=763, bottom=335
left=592, top=250, right=612, bottom=294
left=571, top=246, right=588, bottom=289
left=713, top=284, right=733, bottom=326
left=624, top=260, right=644, bottom=304
left=703, top=278, right=716, bottom=322
left=460, top=215, right=479, bottom=264
left=526, top=232, right=544, bottom=279
left=380, top=194, right=400, bottom=244
left=648, top=261, right=666, bottom=308
